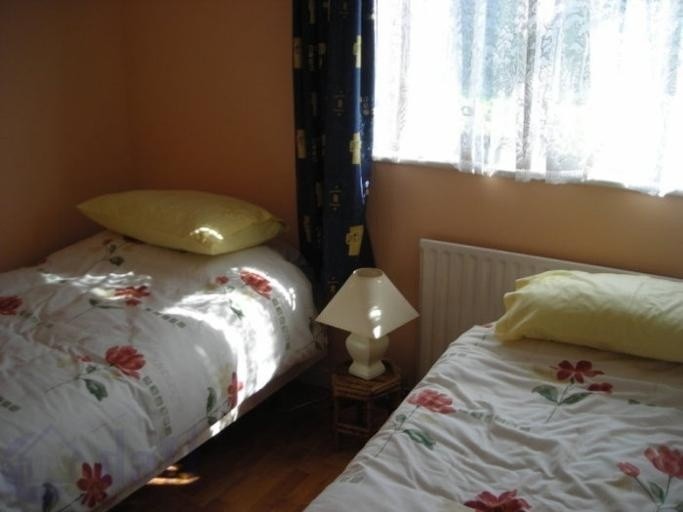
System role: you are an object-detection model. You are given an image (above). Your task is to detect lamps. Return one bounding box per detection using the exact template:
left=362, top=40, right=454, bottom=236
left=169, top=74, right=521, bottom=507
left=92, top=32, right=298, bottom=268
left=314, top=269, right=421, bottom=380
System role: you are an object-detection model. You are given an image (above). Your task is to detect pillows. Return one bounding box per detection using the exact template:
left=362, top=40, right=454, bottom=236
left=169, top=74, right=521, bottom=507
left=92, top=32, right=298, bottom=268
left=492, top=268, right=682, bottom=364
left=75, top=187, right=287, bottom=257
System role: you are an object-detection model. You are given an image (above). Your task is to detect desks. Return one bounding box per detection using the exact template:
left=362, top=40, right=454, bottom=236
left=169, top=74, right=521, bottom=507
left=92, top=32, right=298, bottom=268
left=330, top=362, right=406, bottom=453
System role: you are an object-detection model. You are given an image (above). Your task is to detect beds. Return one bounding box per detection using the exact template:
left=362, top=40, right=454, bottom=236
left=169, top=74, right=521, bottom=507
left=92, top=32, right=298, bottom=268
left=0, top=227, right=329, bottom=512
left=302, top=324, right=681, bottom=512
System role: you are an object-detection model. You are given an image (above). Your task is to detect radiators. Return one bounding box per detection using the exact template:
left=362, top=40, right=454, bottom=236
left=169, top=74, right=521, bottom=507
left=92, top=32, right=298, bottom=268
left=418, top=236, right=624, bottom=377
